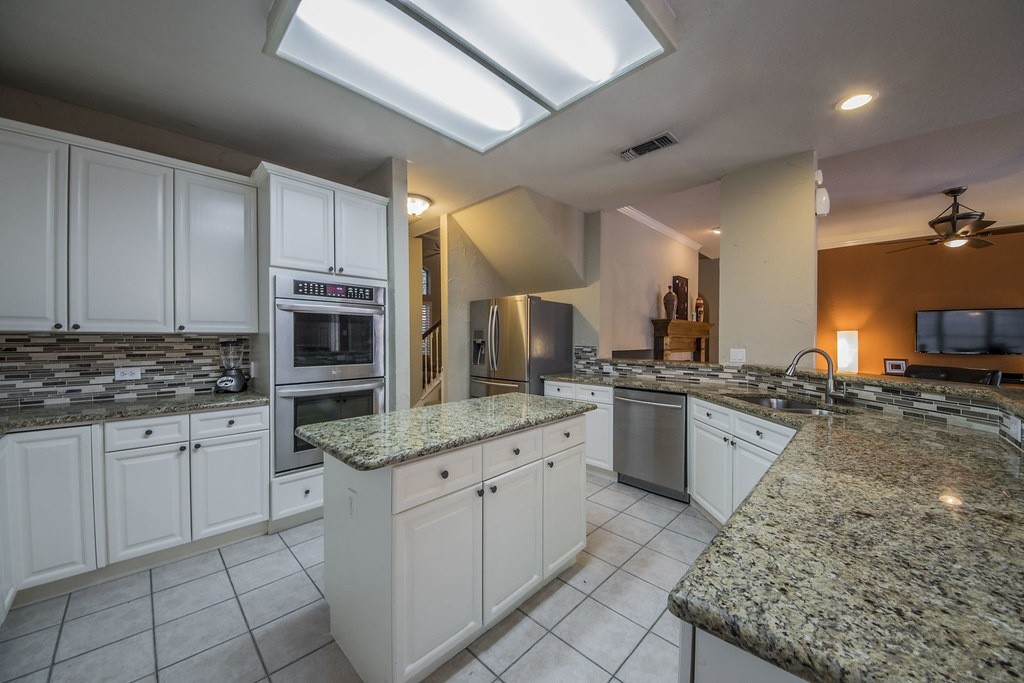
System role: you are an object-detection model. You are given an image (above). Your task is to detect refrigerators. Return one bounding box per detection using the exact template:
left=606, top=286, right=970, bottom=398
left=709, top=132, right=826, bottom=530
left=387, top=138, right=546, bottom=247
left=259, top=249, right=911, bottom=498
left=469, top=295, right=572, bottom=400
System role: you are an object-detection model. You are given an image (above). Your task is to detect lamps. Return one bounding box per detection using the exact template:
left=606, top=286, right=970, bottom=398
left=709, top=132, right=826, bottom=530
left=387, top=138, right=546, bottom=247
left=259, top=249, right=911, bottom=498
left=944, top=237, right=969, bottom=248
left=406, top=193, right=433, bottom=216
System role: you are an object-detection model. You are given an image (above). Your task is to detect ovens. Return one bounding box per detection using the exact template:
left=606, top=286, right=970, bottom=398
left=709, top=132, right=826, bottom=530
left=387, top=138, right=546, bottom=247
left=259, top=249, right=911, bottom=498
left=274, top=276, right=385, bottom=474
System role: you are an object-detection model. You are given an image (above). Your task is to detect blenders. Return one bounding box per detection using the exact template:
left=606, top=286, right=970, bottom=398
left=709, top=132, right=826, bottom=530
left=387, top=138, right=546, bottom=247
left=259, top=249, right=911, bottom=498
left=215, top=341, right=247, bottom=393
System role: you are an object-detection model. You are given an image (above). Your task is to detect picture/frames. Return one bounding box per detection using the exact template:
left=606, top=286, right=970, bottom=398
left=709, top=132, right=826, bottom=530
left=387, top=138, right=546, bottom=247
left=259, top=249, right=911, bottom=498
left=883, top=358, right=908, bottom=376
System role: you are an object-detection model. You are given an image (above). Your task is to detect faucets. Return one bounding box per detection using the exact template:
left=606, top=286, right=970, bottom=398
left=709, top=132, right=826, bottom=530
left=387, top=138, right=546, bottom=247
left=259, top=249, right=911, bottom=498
left=784, top=347, right=849, bottom=405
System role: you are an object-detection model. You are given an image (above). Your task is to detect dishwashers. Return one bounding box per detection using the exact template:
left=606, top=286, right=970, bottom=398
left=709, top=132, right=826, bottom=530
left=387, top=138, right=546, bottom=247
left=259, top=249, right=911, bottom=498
left=612, top=388, right=691, bottom=504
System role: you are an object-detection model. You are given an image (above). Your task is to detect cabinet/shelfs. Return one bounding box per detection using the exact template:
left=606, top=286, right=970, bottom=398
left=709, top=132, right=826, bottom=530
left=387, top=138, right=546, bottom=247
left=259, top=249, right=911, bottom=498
left=545, top=380, right=615, bottom=472
left=0, top=424, right=99, bottom=626
left=98, top=405, right=271, bottom=569
left=542, top=414, right=587, bottom=582
left=69, top=141, right=257, bottom=331
left=255, top=160, right=394, bottom=281
left=687, top=398, right=796, bottom=525
left=0, top=117, right=69, bottom=332
left=392, top=432, right=543, bottom=682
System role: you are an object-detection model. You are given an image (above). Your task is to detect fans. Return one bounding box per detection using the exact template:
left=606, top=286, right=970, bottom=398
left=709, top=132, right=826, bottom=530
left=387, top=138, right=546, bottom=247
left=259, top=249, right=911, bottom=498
left=887, top=182, right=997, bottom=254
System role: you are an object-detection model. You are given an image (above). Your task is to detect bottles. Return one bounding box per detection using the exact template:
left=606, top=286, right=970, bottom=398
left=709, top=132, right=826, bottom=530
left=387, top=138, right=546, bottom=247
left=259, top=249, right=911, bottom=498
left=695, top=296, right=704, bottom=322
left=664, top=285, right=678, bottom=320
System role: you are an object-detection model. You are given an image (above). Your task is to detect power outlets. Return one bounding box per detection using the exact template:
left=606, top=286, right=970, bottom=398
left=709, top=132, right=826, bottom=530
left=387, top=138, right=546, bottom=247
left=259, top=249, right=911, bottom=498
left=115, top=367, right=141, bottom=381
left=346, top=487, right=358, bottom=526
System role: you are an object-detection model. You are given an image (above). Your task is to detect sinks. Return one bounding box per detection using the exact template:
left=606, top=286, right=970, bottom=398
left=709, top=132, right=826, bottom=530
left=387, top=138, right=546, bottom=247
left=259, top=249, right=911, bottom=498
left=779, top=409, right=849, bottom=415
left=719, top=393, right=819, bottom=409
